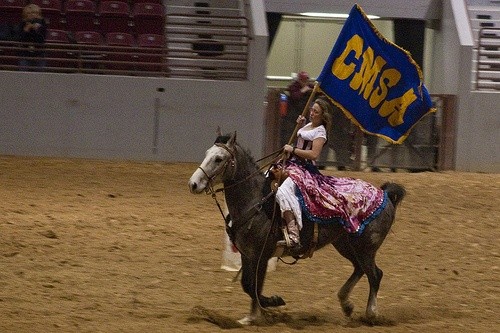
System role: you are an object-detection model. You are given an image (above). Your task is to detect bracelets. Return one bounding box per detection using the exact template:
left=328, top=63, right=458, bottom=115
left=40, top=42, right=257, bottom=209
left=292, top=147, right=297, bottom=155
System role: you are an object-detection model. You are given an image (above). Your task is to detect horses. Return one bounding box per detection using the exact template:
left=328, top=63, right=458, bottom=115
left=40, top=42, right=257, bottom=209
left=186, top=123, right=409, bottom=328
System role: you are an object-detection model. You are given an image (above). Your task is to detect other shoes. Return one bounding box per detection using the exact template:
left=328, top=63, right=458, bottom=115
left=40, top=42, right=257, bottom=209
left=318, top=165, right=325, bottom=170
left=339, top=165, right=347, bottom=170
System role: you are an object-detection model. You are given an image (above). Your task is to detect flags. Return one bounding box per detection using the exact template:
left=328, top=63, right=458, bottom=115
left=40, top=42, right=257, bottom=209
left=313, top=3, right=437, bottom=144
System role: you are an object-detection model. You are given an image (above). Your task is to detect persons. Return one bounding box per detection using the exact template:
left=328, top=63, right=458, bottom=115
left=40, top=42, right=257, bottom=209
left=290, top=71, right=316, bottom=98
left=12, top=6, right=50, bottom=72
left=274, top=98, right=332, bottom=248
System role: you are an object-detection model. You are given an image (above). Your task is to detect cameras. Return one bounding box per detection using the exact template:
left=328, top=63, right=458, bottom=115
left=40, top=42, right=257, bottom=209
left=30, top=18, right=39, bottom=24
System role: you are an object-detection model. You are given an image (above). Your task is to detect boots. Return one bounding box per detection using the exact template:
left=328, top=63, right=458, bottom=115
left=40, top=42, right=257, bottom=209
left=277, top=209, right=301, bottom=248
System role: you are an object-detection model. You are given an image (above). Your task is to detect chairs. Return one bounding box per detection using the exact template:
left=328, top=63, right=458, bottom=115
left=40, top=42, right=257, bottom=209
left=0, top=0, right=167, bottom=77
